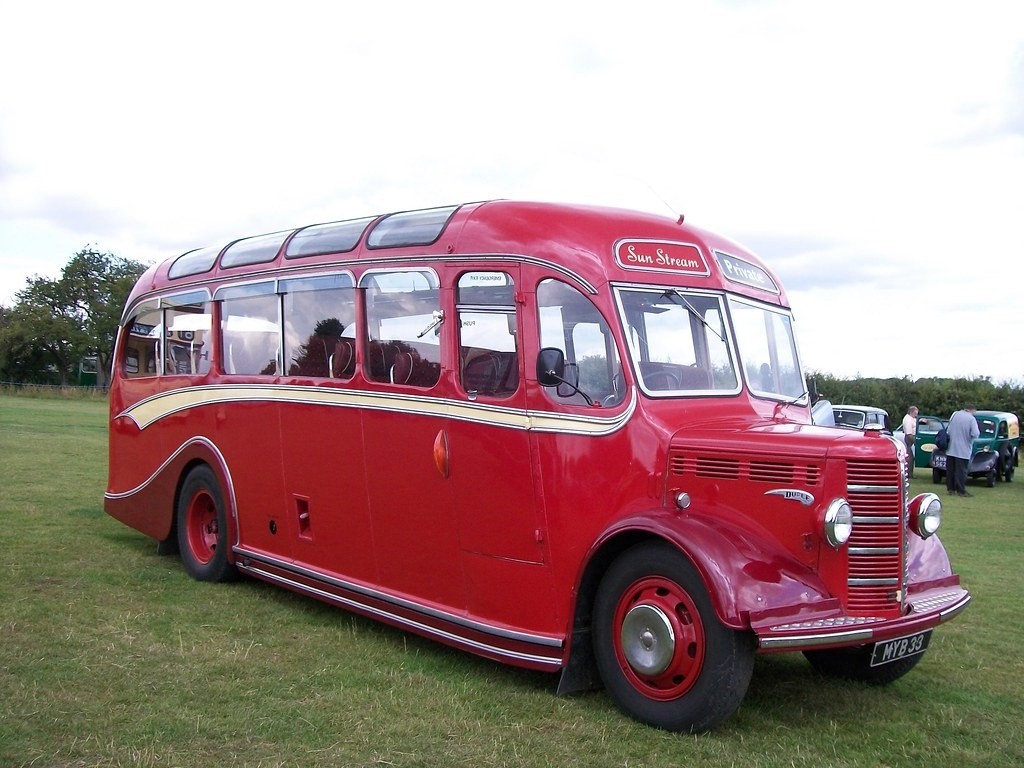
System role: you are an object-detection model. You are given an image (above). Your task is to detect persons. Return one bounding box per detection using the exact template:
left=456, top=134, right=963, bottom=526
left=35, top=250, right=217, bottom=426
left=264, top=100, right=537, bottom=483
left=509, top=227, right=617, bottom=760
left=935, top=403, right=980, bottom=498
left=902, top=406, right=928, bottom=480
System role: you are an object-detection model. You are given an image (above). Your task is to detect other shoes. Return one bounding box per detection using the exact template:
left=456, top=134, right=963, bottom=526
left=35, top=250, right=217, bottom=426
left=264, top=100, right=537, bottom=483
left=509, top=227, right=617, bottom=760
left=959, top=492, right=974, bottom=497
left=948, top=489, right=955, bottom=494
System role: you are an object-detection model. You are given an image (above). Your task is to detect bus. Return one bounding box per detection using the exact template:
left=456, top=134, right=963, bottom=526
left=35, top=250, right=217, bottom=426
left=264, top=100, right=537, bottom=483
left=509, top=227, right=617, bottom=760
left=103, top=197, right=976, bottom=735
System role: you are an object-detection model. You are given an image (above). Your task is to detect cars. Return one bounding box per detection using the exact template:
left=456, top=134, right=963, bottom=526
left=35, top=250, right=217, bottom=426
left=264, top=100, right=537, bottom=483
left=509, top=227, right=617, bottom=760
left=891, top=417, right=950, bottom=458
left=831, top=405, right=893, bottom=437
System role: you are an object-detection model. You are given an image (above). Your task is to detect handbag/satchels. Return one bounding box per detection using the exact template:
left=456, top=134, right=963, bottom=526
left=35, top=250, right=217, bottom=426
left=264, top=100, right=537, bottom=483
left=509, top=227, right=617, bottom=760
left=935, top=428, right=950, bottom=451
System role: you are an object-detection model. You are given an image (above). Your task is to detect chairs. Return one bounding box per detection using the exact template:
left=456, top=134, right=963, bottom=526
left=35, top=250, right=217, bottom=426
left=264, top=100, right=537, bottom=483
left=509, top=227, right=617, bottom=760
left=198, top=331, right=713, bottom=409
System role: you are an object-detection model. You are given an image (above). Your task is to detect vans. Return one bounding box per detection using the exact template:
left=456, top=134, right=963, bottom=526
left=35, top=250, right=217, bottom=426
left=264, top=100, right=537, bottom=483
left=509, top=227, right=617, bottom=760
left=914, top=410, right=1020, bottom=487
